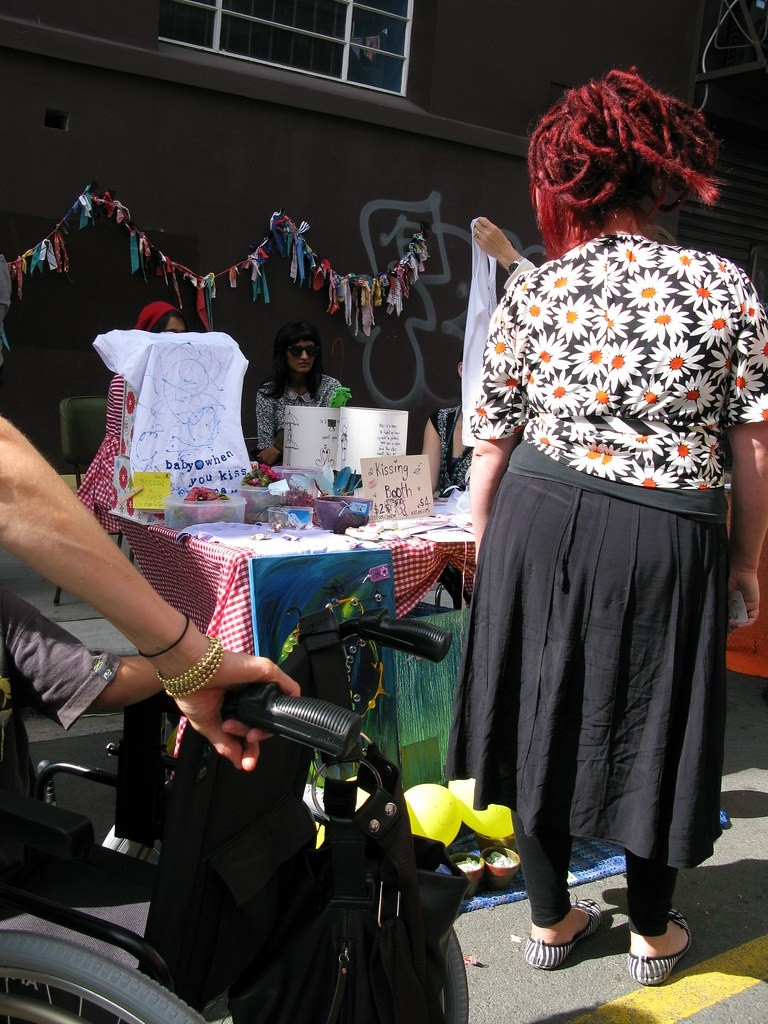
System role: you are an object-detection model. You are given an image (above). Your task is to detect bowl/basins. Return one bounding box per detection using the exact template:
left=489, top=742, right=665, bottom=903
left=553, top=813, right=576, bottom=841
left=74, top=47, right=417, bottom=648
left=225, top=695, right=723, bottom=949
left=238, top=486, right=287, bottom=524
left=164, top=495, right=247, bottom=529
left=314, top=496, right=373, bottom=530
left=272, top=465, right=319, bottom=489
left=267, top=505, right=314, bottom=532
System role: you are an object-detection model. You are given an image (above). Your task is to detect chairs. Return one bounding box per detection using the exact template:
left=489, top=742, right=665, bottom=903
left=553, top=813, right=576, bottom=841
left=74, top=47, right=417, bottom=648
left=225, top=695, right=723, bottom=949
left=53, top=395, right=135, bottom=606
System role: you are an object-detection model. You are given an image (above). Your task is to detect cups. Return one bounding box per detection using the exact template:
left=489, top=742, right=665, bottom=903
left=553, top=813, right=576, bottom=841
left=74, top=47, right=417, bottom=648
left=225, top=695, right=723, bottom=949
left=440, top=853, right=484, bottom=897
left=481, top=846, right=521, bottom=890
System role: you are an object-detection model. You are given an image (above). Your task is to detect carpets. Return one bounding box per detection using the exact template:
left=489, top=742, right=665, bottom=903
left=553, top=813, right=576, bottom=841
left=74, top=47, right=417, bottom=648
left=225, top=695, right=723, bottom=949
left=439, top=808, right=730, bottom=913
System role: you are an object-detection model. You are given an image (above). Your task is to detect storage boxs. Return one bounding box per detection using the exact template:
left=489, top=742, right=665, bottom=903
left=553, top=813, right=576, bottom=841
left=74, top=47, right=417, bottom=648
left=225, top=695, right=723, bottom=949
left=164, top=467, right=322, bottom=530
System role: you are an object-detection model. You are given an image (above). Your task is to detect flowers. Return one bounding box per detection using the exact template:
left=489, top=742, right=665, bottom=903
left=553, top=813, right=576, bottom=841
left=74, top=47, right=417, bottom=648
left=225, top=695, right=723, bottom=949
left=182, top=487, right=232, bottom=522
left=285, top=486, right=315, bottom=507
left=239, top=462, right=282, bottom=488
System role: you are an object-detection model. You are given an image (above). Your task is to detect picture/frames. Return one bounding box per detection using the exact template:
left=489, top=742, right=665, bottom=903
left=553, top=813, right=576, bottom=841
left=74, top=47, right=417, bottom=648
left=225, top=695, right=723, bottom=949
left=248, top=548, right=405, bottom=793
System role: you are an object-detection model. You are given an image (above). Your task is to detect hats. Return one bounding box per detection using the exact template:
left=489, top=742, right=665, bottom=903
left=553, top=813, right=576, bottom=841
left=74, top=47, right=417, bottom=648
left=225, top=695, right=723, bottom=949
left=134, top=300, right=182, bottom=332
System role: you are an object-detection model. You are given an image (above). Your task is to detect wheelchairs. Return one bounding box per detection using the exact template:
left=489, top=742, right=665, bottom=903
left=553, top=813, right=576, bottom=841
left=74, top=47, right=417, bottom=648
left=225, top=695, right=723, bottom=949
left=1, top=601, right=468, bottom=1024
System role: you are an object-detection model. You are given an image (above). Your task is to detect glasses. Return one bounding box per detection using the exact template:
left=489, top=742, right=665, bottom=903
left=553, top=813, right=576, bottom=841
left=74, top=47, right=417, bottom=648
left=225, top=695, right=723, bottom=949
left=286, top=344, right=319, bottom=357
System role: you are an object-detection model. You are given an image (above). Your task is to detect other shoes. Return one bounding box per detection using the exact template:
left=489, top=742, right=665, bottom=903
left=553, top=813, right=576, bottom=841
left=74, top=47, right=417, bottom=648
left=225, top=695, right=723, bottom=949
left=525, top=895, right=601, bottom=971
left=628, top=907, right=691, bottom=985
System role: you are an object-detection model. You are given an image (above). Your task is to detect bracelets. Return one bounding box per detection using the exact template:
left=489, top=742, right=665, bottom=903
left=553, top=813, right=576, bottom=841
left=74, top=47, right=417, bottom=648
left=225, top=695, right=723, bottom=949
left=154, top=635, right=223, bottom=697
left=138, top=611, right=190, bottom=658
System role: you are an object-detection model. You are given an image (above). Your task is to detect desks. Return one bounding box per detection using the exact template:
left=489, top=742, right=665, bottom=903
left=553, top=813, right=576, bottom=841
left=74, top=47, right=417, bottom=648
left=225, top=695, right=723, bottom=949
left=105, top=501, right=475, bottom=795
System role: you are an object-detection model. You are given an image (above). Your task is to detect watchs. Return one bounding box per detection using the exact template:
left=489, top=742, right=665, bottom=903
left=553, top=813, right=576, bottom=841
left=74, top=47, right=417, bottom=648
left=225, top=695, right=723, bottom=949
left=507, top=256, right=525, bottom=276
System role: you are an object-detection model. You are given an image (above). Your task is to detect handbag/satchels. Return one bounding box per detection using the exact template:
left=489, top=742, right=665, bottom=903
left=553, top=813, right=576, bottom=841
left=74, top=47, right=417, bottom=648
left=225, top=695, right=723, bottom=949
left=141, top=608, right=470, bottom=1021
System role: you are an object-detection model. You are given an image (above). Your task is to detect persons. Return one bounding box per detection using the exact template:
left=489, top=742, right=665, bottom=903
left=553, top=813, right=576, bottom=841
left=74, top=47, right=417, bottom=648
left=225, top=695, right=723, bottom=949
left=74, top=301, right=194, bottom=532
left=439, top=66, right=768, bottom=988
left=0, top=413, right=301, bottom=801
left=255, top=316, right=342, bottom=466
left=420, top=352, right=476, bottom=611
